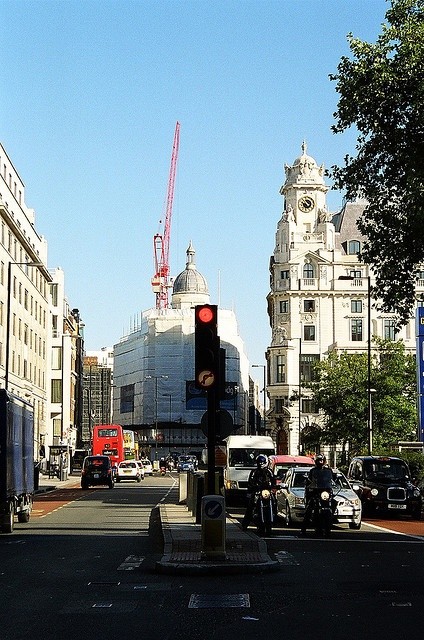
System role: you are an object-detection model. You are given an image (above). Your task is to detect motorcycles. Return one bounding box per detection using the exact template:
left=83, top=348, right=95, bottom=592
left=247, top=483, right=281, bottom=534
left=167, top=462, right=174, bottom=472
left=160, top=467, right=165, bottom=475
left=303, top=473, right=343, bottom=536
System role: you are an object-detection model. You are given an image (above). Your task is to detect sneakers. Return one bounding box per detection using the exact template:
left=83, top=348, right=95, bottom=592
left=300, top=529, right=306, bottom=535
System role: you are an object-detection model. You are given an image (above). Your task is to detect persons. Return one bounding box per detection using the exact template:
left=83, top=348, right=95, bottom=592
left=298, top=454, right=333, bottom=537
left=240, top=453, right=277, bottom=528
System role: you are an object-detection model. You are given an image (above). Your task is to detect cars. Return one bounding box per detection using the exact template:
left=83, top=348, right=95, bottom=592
left=134, top=461, right=144, bottom=480
left=139, top=460, right=152, bottom=476
left=275, top=467, right=362, bottom=529
left=116, top=461, right=141, bottom=482
left=346, top=456, right=421, bottom=519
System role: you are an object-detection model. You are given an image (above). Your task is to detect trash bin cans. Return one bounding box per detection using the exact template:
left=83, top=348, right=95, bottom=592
left=61, top=469, right=68, bottom=481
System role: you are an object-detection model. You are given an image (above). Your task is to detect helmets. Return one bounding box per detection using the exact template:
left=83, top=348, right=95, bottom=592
left=256, top=454, right=268, bottom=468
left=314, top=453, right=326, bottom=468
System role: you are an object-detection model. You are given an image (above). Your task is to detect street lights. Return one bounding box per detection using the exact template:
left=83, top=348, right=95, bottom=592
left=162, top=393, right=171, bottom=453
left=283, top=338, right=301, bottom=455
left=5, top=260, right=43, bottom=386
left=251, top=365, right=265, bottom=435
left=337, top=271, right=371, bottom=455
left=143, top=375, right=168, bottom=460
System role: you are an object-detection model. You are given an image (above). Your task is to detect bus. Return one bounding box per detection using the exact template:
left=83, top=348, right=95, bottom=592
left=92, top=425, right=124, bottom=476
left=122, top=430, right=139, bottom=461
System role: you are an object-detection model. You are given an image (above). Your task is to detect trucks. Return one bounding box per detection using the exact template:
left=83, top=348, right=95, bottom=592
left=0, top=388, right=34, bottom=533
left=222, top=435, right=275, bottom=507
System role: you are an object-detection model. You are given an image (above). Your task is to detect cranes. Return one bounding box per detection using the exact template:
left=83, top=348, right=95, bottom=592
left=150, top=121, right=179, bottom=307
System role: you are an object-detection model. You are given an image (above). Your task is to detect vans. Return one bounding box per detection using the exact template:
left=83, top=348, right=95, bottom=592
left=80, top=456, right=114, bottom=489
left=267, top=455, right=315, bottom=494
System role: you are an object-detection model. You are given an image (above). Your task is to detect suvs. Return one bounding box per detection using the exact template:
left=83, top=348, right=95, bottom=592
left=175, top=454, right=198, bottom=473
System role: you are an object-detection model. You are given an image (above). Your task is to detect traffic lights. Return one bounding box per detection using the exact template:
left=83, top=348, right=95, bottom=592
left=194, top=304, right=219, bottom=369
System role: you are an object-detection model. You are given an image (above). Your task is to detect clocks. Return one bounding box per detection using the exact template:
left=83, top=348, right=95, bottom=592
left=297, top=196, right=315, bottom=214
